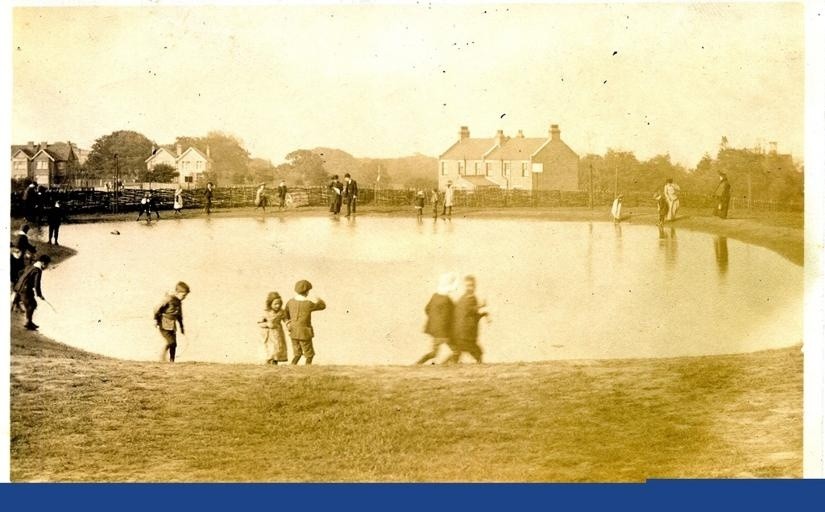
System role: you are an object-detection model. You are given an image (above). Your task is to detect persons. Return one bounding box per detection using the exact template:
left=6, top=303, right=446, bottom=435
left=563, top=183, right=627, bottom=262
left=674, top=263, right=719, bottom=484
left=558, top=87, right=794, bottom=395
left=664, top=179, right=680, bottom=220
left=10, top=181, right=64, bottom=330
left=413, top=179, right=454, bottom=218
left=713, top=173, right=730, bottom=219
left=257, top=291, right=288, bottom=365
left=448, top=275, right=488, bottom=364
left=416, top=272, right=456, bottom=365
left=611, top=194, right=625, bottom=225
left=284, top=280, right=326, bottom=364
left=153, top=281, right=190, bottom=363
left=136, top=172, right=358, bottom=222
left=652, top=187, right=669, bottom=223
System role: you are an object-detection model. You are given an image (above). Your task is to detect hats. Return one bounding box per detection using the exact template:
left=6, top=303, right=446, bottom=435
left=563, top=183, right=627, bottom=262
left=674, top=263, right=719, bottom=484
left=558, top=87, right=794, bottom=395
left=295, top=280, right=312, bottom=294
